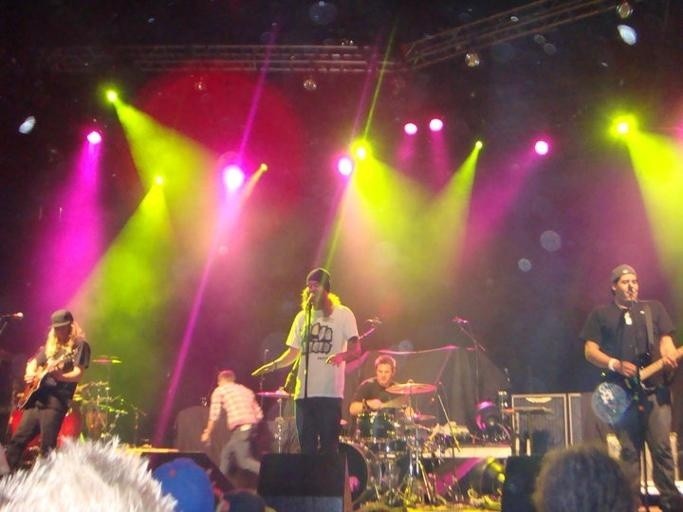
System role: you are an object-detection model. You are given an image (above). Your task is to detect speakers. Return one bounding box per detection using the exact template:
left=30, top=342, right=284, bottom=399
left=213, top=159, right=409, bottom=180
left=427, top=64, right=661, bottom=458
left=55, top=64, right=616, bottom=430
left=500, top=453, right=541, bottom=511
left=257, top=449, right=343, bottom=497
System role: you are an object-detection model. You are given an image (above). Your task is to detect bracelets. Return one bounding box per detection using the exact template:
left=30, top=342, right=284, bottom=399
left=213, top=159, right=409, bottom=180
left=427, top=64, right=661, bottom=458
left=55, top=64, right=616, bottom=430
left=272, top=360, right=279, bottom=371
left=608, top=358, right=617, bottom=373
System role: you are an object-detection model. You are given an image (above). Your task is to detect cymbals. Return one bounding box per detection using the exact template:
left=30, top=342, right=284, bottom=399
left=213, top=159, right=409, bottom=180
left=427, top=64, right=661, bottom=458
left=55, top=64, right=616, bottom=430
left=386, top=382, right=434, bottom=397
left=255, top=391, right=295, bottom=400
left=409, top=412, right=433, bottom=422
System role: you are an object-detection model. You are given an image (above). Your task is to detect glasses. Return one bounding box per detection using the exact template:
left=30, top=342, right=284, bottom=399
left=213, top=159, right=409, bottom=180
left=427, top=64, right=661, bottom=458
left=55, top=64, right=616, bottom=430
left=306, top=282, right=322, bottom=289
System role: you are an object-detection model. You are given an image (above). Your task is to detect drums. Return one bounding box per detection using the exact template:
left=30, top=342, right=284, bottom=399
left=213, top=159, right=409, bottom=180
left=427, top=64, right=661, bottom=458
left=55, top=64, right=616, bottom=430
left=350, top=413, right=403, bottom=442
left=435, top=432, right=455, bottom=450
left=406, top=425, right=431, bottom=446
left=386, top=437, right=407, bottom=455
left=339, top=439, right=385, bottom=512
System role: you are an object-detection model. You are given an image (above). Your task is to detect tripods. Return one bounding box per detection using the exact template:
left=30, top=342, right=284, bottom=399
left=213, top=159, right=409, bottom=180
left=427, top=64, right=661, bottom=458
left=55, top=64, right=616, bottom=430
left=385, top=396, right=438, bottom=505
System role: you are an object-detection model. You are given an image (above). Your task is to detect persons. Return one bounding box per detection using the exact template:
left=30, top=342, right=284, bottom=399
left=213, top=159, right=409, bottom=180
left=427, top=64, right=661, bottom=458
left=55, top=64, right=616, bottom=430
left=579, top=263, right=683, bottom=512
left=148, top=456, right=213, bottom=512
left=533, top=444, right=640, bottom=512
left=6, top=309, right=91, bottom=472
left=201, top=369, right=264, bottom=477
left=251, top=268, right=361, bottom=454
left=350, top=355, right=412, bottom=507
left=0, top=433, right=176, bottom=512
left=218, top=490, right=267, bottom=512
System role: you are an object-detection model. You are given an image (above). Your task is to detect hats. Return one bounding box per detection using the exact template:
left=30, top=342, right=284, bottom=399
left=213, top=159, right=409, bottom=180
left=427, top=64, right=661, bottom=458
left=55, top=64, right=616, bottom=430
left=305, top=268, right=331, bottom=293
left=47, top=309, right=75, bottom=329
left=610, top=263, right=638, bottom=283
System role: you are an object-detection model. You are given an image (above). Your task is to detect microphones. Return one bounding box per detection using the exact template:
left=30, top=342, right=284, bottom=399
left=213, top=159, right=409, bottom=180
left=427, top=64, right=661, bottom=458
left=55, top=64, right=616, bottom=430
left=303, top=293, right=314, bottom=309
left=1, top=312, right=24, bottom=322
left=366, top=317, right=384, bottom=326
left=452, top=316, right=469, bottom=328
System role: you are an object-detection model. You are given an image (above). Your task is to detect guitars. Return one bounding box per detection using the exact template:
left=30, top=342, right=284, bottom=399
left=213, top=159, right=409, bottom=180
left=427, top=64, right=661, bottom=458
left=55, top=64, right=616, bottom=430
left=17, top=351, right=72, bottom=412
left=591, top=347, right=683, bottom=425
